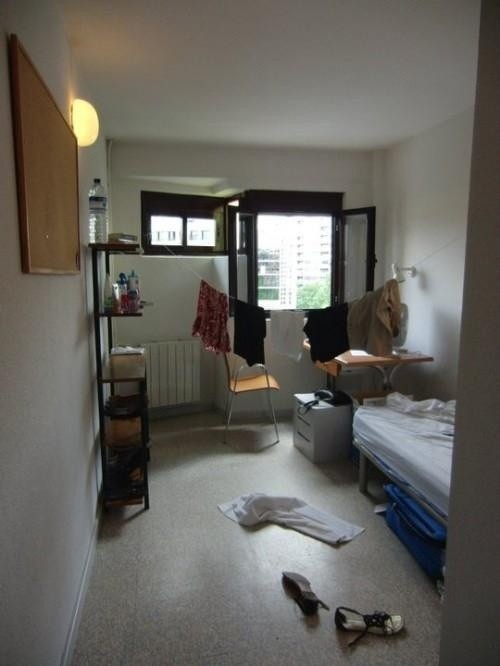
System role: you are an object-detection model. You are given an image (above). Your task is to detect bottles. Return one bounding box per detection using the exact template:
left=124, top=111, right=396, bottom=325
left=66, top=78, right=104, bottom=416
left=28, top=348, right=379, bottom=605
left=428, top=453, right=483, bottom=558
left=127, top=269, right=142, bottom=312
left=83, top=175, right=109, bottom=246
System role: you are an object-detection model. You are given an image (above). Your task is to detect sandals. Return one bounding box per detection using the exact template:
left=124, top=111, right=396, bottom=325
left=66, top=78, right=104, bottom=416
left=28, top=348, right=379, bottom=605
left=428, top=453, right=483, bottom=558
left=335, top=606, right=403, bottom=635
left=282, top=572, right=318, bottom=615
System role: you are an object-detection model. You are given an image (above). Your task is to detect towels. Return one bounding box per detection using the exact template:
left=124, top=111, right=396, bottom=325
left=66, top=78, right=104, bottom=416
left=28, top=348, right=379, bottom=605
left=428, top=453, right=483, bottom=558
left=218, top=492, right=366, bottom=546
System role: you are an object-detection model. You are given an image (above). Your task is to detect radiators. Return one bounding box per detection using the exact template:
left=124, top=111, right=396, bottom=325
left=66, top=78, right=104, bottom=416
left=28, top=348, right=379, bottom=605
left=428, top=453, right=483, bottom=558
left=140, top=341, right=201, bottom=407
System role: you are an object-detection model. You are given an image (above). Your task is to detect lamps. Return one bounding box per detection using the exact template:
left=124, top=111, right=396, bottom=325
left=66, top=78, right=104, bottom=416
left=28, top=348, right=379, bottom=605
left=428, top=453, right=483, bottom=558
left=391, top=263, right=421, bottom=287
left=72, top=98, right=100, bottom=147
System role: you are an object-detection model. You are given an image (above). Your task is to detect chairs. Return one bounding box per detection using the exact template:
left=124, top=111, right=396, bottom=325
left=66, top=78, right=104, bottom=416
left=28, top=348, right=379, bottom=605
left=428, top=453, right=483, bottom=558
left=224, top=352, right=281, bottom=444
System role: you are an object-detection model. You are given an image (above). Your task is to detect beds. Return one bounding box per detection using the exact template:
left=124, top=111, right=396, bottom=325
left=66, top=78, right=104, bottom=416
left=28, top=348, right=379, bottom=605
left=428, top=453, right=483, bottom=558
left=354, top=406, right=456, bottom=530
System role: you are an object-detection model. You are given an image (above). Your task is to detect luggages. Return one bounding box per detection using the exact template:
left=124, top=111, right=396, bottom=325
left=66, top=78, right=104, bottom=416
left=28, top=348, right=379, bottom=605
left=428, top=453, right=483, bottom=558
left=382, top=482, right=446, bottom=577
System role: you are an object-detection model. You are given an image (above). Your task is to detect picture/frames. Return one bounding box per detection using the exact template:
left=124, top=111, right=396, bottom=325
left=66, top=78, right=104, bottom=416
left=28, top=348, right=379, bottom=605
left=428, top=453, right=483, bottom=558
left=10, top=34, right=81, bottom=273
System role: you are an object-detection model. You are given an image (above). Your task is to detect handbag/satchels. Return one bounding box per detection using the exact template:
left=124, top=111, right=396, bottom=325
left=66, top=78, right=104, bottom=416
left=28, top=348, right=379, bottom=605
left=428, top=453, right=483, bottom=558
left=298, top=390, right=352, bottom=415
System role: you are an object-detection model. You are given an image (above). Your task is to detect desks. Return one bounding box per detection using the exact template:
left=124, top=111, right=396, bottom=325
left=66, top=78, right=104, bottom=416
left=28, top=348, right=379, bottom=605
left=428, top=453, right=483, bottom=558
left=303, top=338, right=434, bottom=393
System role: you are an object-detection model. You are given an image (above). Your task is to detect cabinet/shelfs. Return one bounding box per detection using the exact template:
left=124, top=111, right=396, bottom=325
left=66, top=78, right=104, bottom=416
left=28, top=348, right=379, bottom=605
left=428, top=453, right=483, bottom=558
left=293, top=393, right=352, bottom=464
left=89, top=242, right=151, bottom=510
